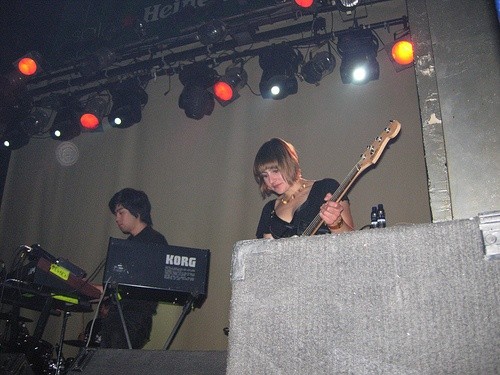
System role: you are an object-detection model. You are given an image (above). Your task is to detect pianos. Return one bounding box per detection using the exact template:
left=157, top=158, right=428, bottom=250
left=0, top=257, right=101, bottom=317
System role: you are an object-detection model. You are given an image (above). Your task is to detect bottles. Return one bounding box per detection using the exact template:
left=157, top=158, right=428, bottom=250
left=371, top=207, right=377, bottom=227
left=377, top=204, right=386, bottom=228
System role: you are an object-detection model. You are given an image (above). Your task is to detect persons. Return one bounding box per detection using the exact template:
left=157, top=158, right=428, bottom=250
left=253, top=136, right=354, bottom=241
left=90, top=187, right=170, bottom=351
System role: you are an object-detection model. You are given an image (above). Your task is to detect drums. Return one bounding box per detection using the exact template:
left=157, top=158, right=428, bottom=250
left=0, top=326, right=75, bottom=375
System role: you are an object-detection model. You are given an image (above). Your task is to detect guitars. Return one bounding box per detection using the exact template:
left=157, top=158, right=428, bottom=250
left=300, top=119, right=402, bottom=237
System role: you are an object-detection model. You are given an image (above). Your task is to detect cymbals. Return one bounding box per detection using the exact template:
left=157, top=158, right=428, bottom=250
left=0, top=312, right=33, bottom=322
left=64, top=339, right=100, bottom=348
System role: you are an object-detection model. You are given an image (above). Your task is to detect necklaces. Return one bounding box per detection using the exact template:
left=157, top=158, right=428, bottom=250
left=280, top=179, right=308, bottom=204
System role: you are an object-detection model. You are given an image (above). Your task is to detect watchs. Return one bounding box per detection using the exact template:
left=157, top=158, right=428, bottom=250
left=329, top=218, right=344, bottom=230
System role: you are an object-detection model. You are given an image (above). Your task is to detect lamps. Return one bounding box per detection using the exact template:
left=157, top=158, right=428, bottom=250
left=0, top=0, right=416, bottom=150
